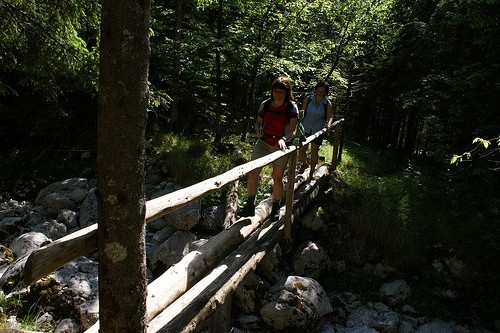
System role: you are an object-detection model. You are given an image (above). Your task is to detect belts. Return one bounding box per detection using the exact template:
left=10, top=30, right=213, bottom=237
left=264, top=132, right=284, bottom=139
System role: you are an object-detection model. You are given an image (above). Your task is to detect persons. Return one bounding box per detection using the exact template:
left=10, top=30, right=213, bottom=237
left=298, top=80, right=333, bottom=180
left=236, top=77, right=299, bottom=221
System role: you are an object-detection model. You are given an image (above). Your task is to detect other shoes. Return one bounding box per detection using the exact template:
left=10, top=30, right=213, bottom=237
left=270, top=204, right=281, bottom=220
left=298, top=161, right=309, bottom=174
left=309, top=171, right=315, bottom=180
left=236, top=204, right=255, bottom=217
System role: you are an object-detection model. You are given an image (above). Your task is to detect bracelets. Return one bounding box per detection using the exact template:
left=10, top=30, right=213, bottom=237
left=282, top=136, right=290, bottom=141
